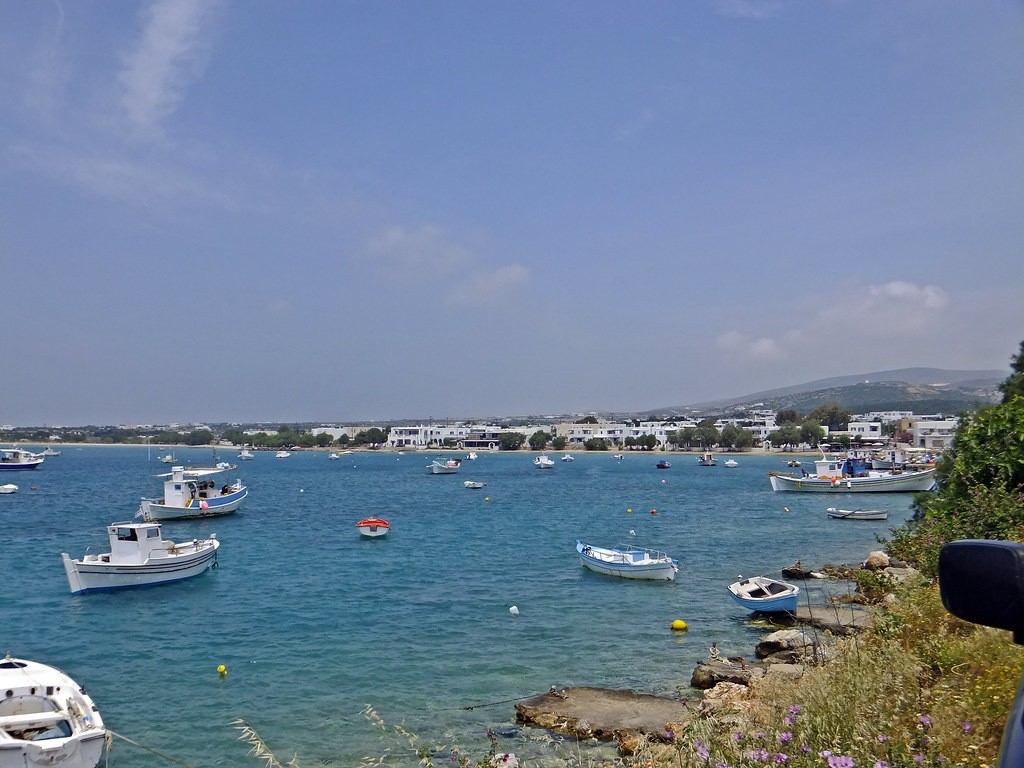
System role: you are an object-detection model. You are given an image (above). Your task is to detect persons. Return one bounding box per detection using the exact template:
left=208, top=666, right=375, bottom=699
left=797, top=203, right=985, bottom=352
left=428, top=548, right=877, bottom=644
left=221, top=485, right=227, bottom=494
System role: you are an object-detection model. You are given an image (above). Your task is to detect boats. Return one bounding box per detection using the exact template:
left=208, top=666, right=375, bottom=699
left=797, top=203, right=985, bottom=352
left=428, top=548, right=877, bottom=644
left=464, top=481, right=486, bottom=490
left=329, top=452, right=341, bottom=462
left=60, top=520, right=225, bottom=593
left=0, top=445, right=47, bottom=471
left=276, top=450, right=291, bottom=459
left=426, top=457, right=464, bottom=474
left=656, top=460, right=673, bottom=469
left=136, top=464, right=249, bottom=519
left=465, top=452, right=477, bottom=460
left=613, top=452, right=624, bottom=460
left=237, top=450, right=254, bottom=461
left=41, top=444, right=63, bottom=458
left=357, top=512, right=391, bottom=539
left=0, top=653, right=107, bottom=768
left=769, top=439, right=941, bottom=493
left=695, top=451, right=721, bottom=467
left=727, top=572, right=800, bottom=612
left=562, top=453, right=576, bottom=466
left=533, top=454, right=556, bottom=470
left=576, top=539, right=679, bottom=582
left=825, top=507, right=889, bottom=520
left=724, top=458, right=739, bottom=469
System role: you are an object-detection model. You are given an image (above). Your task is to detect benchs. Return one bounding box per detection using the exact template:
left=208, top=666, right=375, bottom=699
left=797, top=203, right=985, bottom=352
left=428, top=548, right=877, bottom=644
left=0, top=711, right=69, bottom=726
